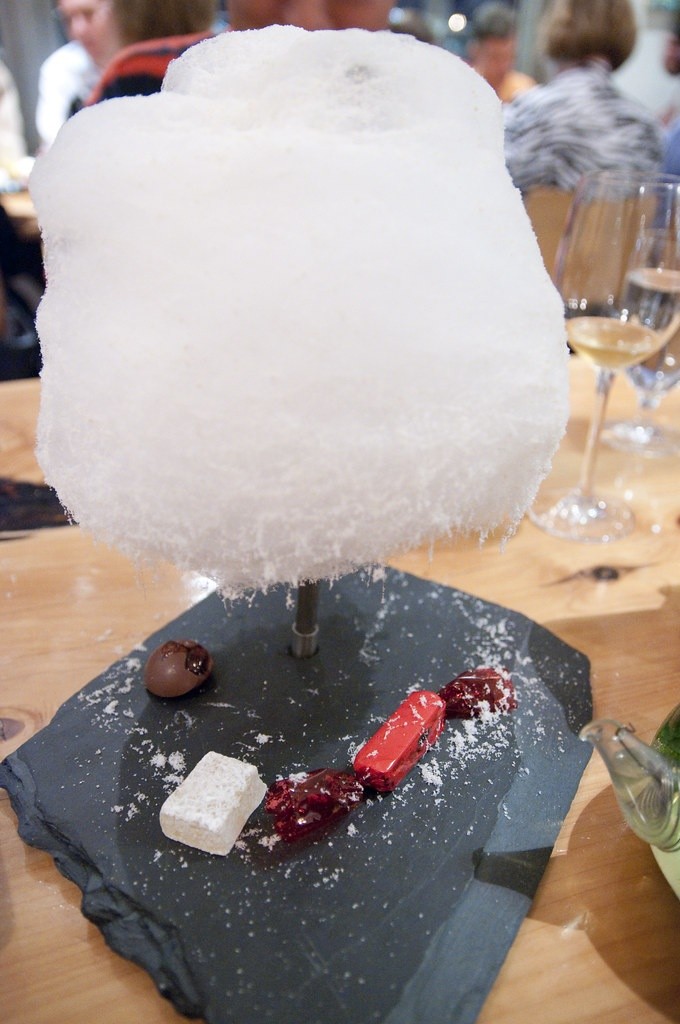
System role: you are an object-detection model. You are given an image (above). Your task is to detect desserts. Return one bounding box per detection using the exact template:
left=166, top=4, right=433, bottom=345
left=143, top=639, right=211, bottom=698
left=160, top=668, right=518, bottom=857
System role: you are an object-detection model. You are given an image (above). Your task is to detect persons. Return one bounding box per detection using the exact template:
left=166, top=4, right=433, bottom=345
left=0, top=0, right=679, bottom=226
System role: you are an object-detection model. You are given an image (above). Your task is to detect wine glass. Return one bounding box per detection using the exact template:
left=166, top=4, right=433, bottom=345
left=599, top=232, right=680, bottom=457
left=525, top=168, right=680, bottom=541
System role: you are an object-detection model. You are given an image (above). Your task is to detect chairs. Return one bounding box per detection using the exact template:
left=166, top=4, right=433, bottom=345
left=526, top=184, right=662, bottom=307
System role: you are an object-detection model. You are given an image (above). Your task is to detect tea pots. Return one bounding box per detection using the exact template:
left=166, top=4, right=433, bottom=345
left=579, top=703, right=680, bottom=897
